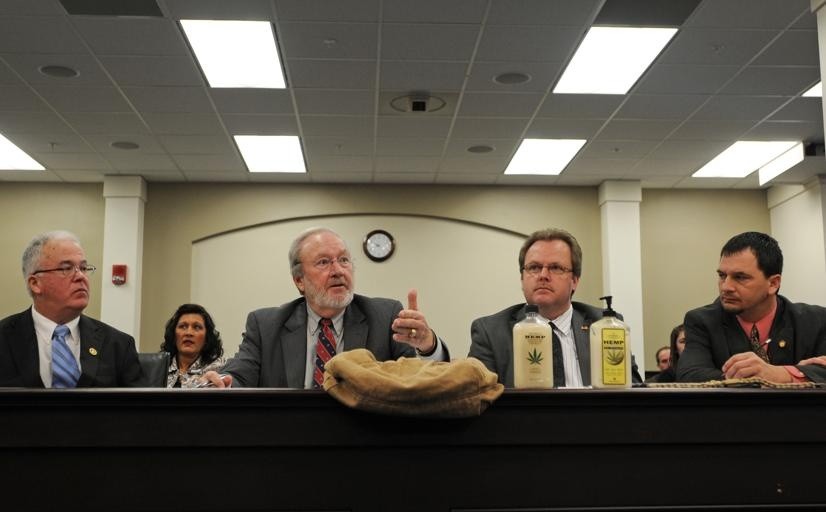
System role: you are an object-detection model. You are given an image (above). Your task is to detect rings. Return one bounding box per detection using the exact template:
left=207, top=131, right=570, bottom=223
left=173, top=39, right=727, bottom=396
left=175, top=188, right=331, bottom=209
left=408, top=328, right=417, bottom=338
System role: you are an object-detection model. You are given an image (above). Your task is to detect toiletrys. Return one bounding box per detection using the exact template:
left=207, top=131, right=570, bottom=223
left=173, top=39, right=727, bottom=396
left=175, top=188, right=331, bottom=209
left=588, top=293, right=632, bottom=391
left=511, top=305, right=555, bottom=390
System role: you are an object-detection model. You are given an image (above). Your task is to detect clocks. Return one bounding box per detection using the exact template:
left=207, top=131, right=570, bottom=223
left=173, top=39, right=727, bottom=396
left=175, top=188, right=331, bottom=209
left=362, top=229, right=395, bottom=263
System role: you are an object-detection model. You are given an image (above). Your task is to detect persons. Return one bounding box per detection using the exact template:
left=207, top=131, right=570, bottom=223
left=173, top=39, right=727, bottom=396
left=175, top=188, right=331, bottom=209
left=157, top=303, right=229, bottom=389
left=675, top=231, right=826, bottom=384
left=0, top=229, right=152, bottom=387
left=671, top=323, right=686, bottom=364
left=466, top=226, right=645, bottom=389
left=193, top=224, right=451, bottom=388
left=655, top=347, right=671, bottom=370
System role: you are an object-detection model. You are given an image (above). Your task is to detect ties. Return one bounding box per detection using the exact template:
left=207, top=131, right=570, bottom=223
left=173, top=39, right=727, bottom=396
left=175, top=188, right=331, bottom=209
left=313, top=319, right=336, bottom=390
left=51, top=325, right=80, bottom=388
left=751, top=325, right=771, bottom=364
left=548, top=322, right=566, bottom=388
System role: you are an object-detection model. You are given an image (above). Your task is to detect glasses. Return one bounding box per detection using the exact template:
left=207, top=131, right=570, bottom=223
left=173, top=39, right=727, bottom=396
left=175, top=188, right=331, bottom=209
left=295, top=255, right=352, bottom=268
left=521, top=263, right=574, bottom=274
left=32, top=263, right=96, bottom=276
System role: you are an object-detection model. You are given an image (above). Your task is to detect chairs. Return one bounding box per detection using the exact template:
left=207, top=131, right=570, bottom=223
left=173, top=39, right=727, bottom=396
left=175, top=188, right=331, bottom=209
left=135, top=345, right=170, bottom=387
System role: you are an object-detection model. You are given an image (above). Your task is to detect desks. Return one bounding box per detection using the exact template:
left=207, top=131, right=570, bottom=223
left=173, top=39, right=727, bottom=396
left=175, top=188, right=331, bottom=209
left=0, top=384, right=826, bottom=512
